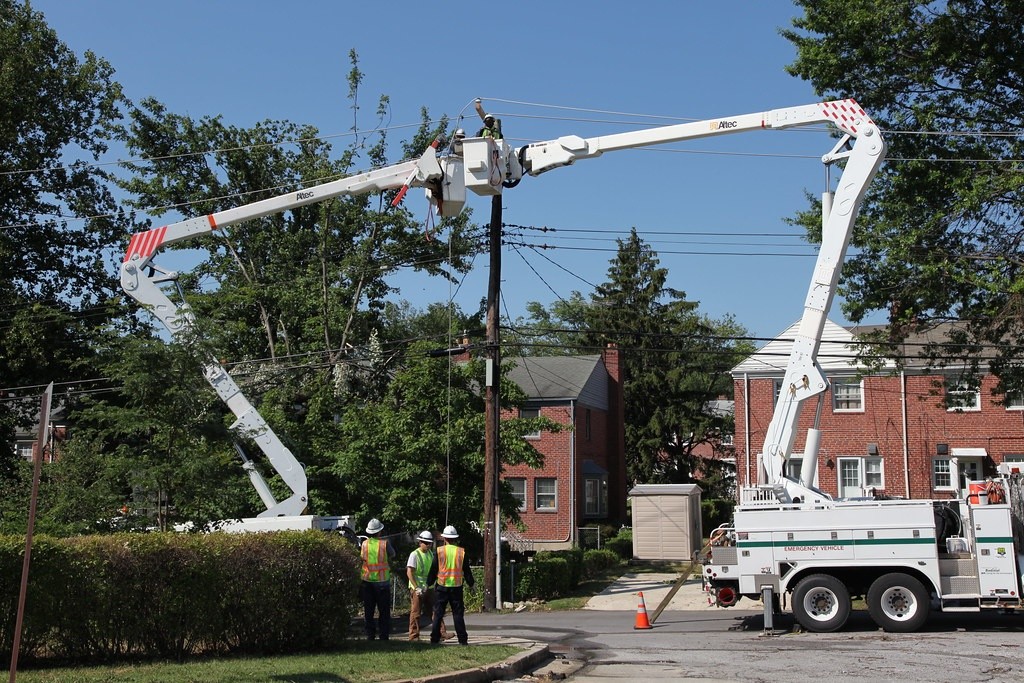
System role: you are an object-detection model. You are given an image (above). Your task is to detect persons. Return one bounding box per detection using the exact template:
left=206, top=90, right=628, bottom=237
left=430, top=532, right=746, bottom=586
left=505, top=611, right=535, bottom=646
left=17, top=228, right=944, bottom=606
left=360, top=519, right=396, bottom=640
left=476, top=113, right=503, bottom=139
left=406, top=531, right=455, bottom=642
left=426, top=526, right=475, bottom=646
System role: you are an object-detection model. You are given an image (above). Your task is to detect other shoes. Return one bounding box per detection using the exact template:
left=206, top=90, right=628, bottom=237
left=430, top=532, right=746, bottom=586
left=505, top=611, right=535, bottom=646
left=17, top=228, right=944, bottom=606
left=431, top=642, right=441, bottom=645
left=460, top=642, right=468, bottom=645
left=440, top=632, right=455, bottom=641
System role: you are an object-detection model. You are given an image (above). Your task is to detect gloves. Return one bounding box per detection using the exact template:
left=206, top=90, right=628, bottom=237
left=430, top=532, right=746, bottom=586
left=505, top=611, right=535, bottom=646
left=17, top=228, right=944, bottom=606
left=471, top=584, right=477, bottom=595
left=416, top=587, right=422, bottom=595
left=422, top=585, right=430, bottom=595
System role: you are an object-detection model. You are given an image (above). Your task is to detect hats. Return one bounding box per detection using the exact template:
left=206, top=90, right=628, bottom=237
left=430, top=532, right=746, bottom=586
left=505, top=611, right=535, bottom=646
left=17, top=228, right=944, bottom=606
left=366, top=518, right=384, bottom=534
left=417, top=530, right=434, bottom=542
left=441, top=525, right=459, bottom=538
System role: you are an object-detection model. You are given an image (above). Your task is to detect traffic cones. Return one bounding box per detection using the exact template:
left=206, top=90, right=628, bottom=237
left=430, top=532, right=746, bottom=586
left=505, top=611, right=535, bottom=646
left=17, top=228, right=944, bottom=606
left=633, top=591, right=653, bottom=629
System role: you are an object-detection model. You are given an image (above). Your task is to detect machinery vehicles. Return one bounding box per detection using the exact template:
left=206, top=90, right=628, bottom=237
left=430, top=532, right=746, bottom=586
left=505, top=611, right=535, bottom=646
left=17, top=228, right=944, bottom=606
left=463, top=100, right=1023, bottom=631
left=79, top=126, right=461, bottom=545
